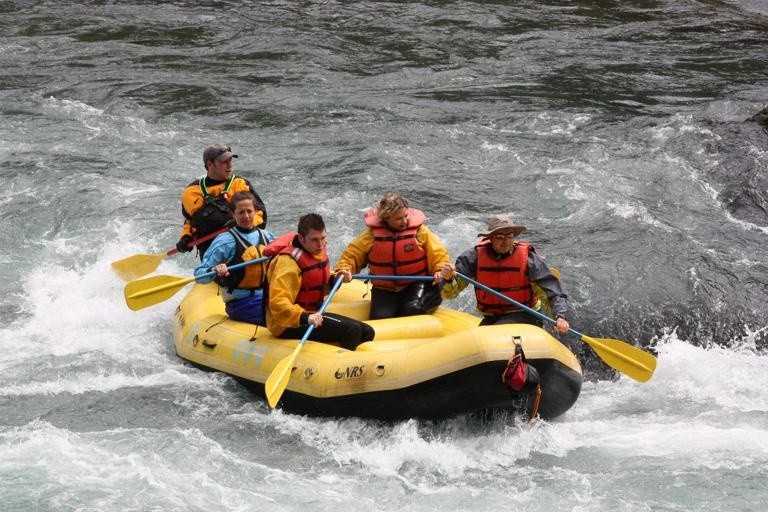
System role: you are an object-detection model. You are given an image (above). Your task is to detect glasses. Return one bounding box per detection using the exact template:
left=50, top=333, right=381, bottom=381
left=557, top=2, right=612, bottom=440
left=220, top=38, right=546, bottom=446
left=492, top=233, right=514, bottom=239
left=210, top=147, right=231, bottom=163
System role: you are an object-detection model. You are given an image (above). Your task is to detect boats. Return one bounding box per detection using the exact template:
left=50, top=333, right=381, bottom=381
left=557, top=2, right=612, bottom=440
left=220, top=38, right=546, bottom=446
left=173, top=271, right=583, bottom=422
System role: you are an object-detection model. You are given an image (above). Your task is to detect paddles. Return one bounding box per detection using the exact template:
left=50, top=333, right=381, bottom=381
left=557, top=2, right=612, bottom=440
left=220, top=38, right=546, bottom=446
left=111, top=211, right=263, bottom=282
left=453, top=271, right=657, bottom=382
left=265, top=274, right=343, bottom=408
left=124, top=256, right=271, bottom=311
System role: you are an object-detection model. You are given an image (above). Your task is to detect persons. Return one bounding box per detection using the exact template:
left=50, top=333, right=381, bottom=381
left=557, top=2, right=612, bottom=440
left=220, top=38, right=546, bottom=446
left=254, top=212, right=376, bottom=347
left=173, top=144, right=265, bottom=254
left=439, top=212, right=570, bottom=338
left=332, top=193, right=461, bottom=322
left=193, top=190, right=280, bottom=324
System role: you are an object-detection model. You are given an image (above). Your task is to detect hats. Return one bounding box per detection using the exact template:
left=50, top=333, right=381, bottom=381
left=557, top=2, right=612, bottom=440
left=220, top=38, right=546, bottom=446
left=478, top=216, right=526, bottom=238
left=203, top=144, right=238, bottom=162
